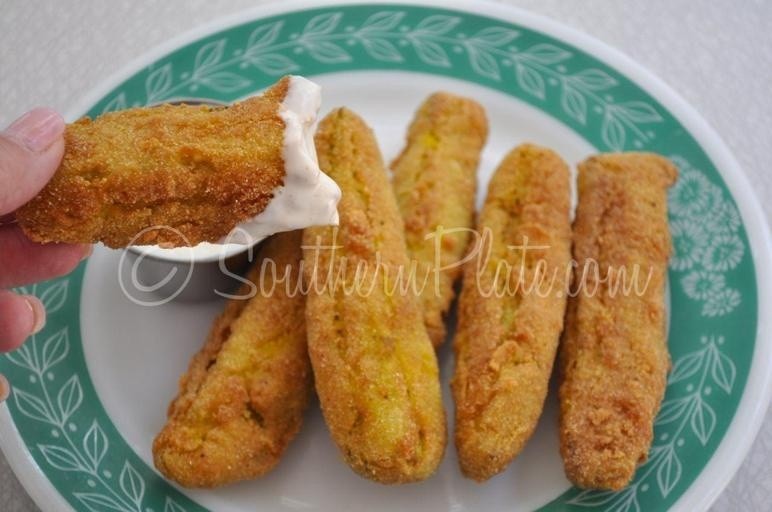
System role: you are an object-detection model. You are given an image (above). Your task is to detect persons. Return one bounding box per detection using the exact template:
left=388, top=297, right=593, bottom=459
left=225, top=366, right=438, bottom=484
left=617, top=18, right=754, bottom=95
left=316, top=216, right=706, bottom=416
left=0, top=109, right=98, bottom=414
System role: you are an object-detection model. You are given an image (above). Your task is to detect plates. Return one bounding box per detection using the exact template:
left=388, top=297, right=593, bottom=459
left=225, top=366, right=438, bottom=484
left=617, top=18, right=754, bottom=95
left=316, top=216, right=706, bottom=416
left=0, top=1, right=768, bottom=512
